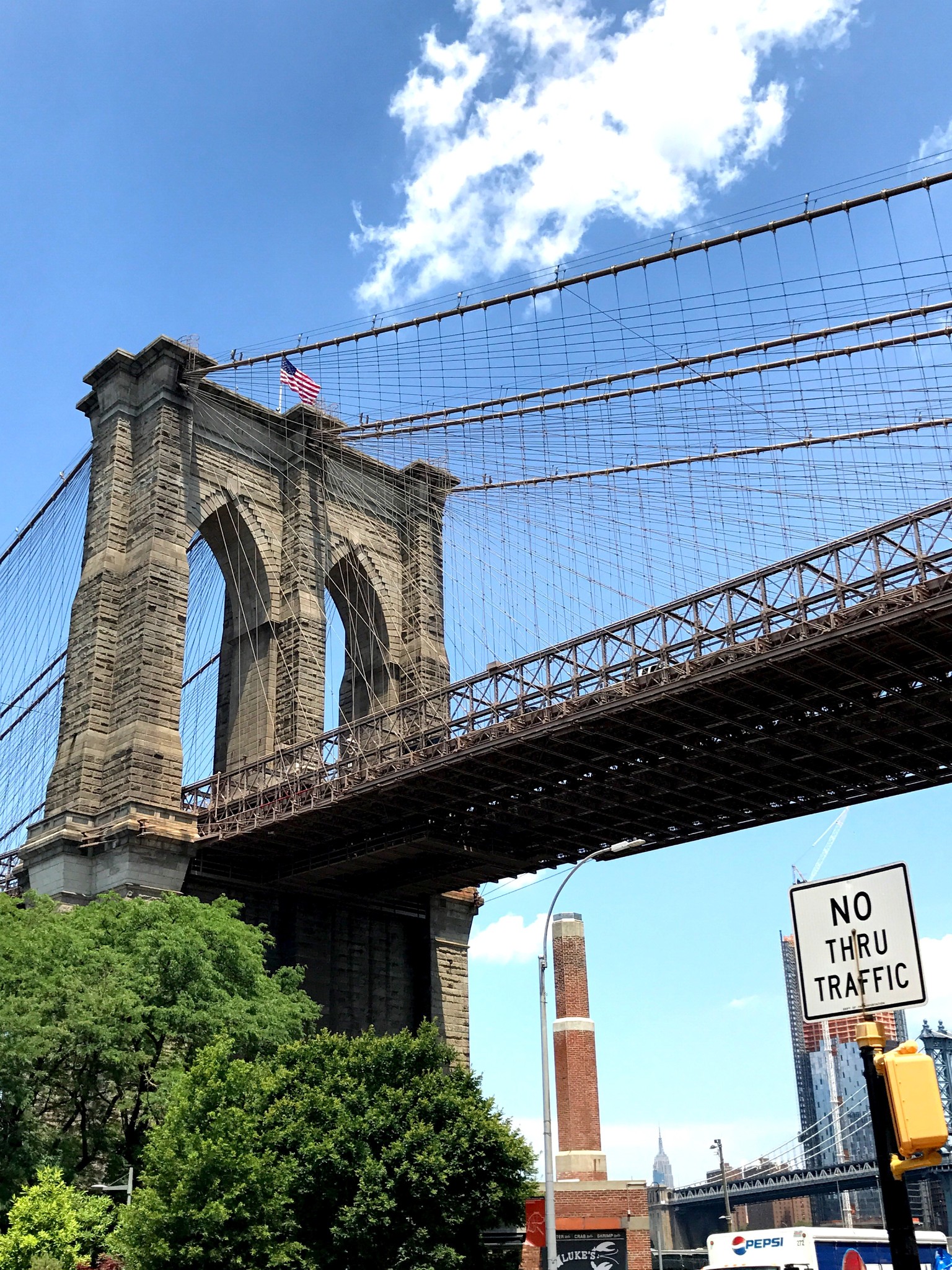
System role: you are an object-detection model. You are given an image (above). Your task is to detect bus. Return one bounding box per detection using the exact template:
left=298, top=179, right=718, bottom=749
left=707, top=1226, right=952, bottom=1270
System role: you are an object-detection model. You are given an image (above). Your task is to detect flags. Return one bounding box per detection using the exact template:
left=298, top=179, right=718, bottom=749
left=279, top=355, right=320, bottom=407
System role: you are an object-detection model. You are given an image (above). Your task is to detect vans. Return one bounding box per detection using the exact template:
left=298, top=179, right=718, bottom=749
left=700, top=1260, right=813, bottom=1270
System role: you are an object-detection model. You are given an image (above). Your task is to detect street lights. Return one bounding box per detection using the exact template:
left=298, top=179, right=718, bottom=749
left=709, top=1138, right=732, bottom=1232
left=538, top=836, right=647, bottom=1270
left=91, top=1166, right=134, bottom=1206
